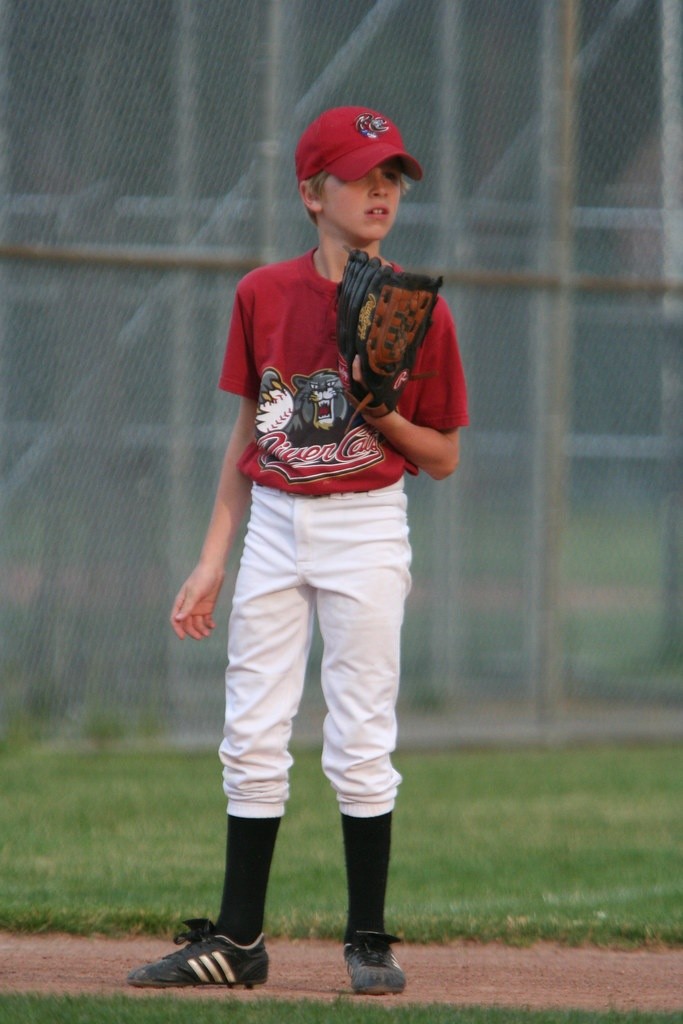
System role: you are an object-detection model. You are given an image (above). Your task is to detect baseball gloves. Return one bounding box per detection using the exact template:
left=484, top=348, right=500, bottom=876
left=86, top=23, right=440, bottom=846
left=335, top=248, right=445, bottom=421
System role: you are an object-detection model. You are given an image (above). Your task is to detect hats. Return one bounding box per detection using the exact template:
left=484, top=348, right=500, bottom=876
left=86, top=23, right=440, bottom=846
left=295, top=106, right=423, bottom=182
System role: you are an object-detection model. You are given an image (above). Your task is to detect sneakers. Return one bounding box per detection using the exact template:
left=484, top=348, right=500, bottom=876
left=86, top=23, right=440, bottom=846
left=125, top=918, right=270, bottom=990
left=343, top=930, right=406, bottom=995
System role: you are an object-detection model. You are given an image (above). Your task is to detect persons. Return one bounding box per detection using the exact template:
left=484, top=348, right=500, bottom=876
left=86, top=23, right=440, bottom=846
left=126, top=107, right=469, bottom=994
